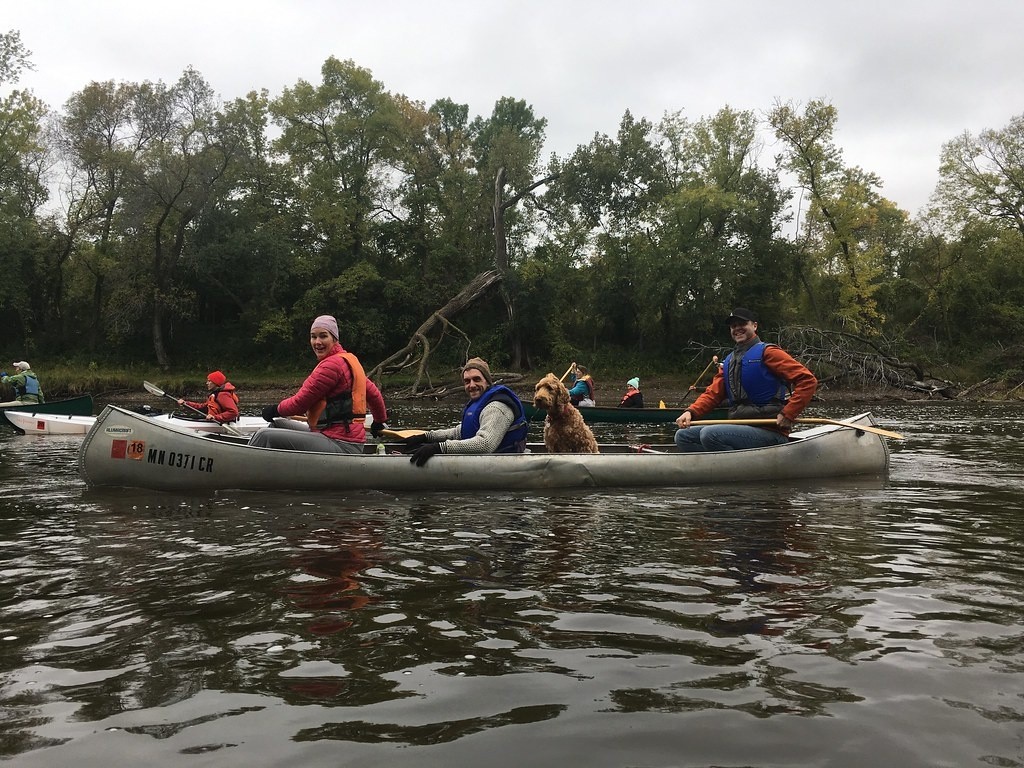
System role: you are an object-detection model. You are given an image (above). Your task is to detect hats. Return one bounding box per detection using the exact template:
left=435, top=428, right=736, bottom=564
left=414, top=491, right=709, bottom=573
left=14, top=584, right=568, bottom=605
left=578, top=365, right=587, bottom=374
left=462, top=357, right=492, bottom=387
left=208, top=371, right=226, bottom=386
left=13, top=361, right=30, bottom=370
left=310, top=315, right=339, bottom=340
left=725, top=308, right=759, bottom=325
left=627, top=377, right=639, bottom=390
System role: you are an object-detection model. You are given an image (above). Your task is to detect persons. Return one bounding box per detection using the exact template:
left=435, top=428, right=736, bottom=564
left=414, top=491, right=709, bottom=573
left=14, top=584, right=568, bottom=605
left=674, top=308, right=817, bottom=453
left=177, top=370, right=239, bottom=424
left=689, top=355, right=729, bottom=407
left=0, top=361, right=45, bottom=404
left=620, top=377, right=644, bottom=408
left=247, top=314, right=387, bottom=454
left=565, top=363, right=595, bottom=407
left=399, top=356, right=528, bottom=467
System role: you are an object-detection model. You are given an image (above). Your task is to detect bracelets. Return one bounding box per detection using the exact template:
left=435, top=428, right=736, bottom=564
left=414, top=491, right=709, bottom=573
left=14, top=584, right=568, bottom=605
left=382, top=423, right=389, bottom=429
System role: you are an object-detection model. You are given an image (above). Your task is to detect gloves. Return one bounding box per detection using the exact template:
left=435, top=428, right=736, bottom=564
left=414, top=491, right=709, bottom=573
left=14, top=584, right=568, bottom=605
left=398, top=434, right=427, bottom=453
left=1, top=372, right=7, bottom=377
left=371, top=420, right=385, bottom=438
left=262, top=403, right=280, bottom=422
left=410, top=442, right=442, bottom=466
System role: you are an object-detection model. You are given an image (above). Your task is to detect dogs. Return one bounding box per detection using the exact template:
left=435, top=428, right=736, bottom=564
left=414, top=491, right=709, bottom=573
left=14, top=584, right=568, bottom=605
left=532, top=372, right=601, bottom=455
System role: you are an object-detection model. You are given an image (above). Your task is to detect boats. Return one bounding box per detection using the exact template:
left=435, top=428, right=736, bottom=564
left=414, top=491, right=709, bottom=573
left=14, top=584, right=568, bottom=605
left=520, top=398, right=731, bottom=425
left=77, top=403, right=890, bottom=494
left=4, top=409, right=310, bottom=434
left=0, top=391, right=95, bottom=426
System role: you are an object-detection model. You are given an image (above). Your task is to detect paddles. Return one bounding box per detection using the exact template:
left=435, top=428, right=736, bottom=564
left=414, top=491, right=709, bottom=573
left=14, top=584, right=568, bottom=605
left=683, top=418, right=906, bottom=440
left=674, top=360, right=714, bottom=408
left=143, top=381, right=243, bottom=436
left=560, top=364, right=573, bottom=382
left=283, top=415, right=428, bottom=438
